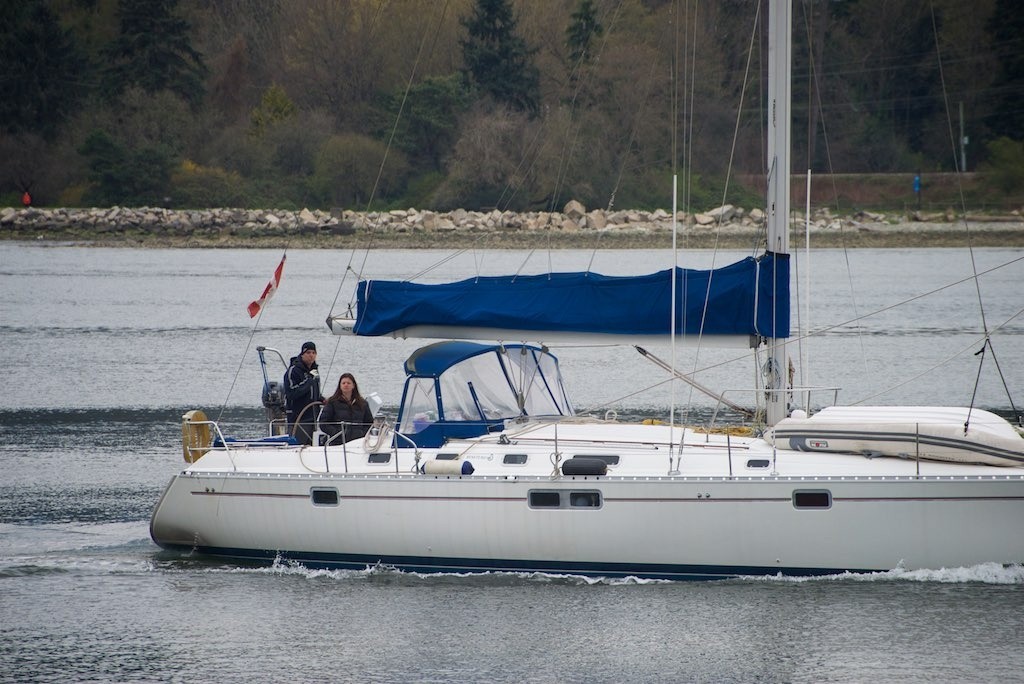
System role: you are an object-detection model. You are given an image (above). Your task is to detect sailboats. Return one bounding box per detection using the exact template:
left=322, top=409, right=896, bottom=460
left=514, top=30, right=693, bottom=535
left=147, top=0, right=1024, bottom=576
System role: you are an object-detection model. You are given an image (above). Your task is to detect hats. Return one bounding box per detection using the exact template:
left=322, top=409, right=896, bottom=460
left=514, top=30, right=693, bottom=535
left=301, top=342, right=315, bottom=354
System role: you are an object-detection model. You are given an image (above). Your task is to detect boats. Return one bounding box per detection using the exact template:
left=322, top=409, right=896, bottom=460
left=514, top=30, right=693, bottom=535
left=761, top=404, right=1024, bottom=468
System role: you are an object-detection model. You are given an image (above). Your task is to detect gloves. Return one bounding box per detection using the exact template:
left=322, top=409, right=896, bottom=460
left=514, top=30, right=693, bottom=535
left=310, top=369, right=319, bottom=379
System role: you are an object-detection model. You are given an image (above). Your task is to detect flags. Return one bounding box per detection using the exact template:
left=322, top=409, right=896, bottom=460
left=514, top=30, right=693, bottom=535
left=246, top=255, right=286, bottom=318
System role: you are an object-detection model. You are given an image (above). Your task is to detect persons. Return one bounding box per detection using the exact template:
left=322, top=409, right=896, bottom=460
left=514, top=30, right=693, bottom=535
left=283, top=342, right=328, bottom=445
left=318, top=373, right=374, bottom=446
